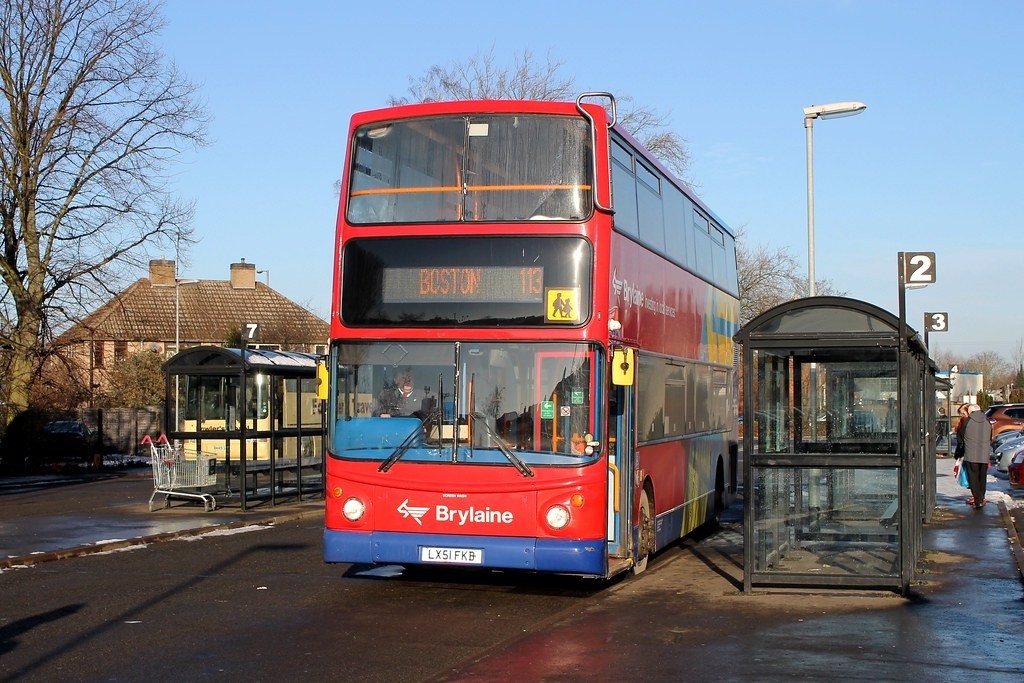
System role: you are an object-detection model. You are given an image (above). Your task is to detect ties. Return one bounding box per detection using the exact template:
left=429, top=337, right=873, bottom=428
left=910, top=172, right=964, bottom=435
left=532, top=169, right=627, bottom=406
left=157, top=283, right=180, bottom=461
left=403, top=392, right=409, bottom=397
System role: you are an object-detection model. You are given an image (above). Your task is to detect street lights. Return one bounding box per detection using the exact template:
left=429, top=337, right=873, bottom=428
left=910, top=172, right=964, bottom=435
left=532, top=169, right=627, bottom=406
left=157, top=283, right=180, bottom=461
left=803, top=102, right=867, bottom=296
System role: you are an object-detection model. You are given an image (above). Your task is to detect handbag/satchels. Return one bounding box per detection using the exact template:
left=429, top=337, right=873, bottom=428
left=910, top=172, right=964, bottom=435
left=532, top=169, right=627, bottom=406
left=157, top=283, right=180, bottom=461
left=953, top=457, right=970, bottom=488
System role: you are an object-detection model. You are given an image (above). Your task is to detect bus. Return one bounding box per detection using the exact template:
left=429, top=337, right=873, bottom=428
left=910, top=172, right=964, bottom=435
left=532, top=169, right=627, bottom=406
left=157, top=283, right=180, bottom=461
left=322, top=91, right=738, bottom=581
left=183, top=373, right=329, bottom=459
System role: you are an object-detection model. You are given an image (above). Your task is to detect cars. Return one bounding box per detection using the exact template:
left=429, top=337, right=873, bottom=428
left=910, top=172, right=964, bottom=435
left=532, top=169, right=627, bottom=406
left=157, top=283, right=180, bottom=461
left=991, top=428, right=1024, bottom=490
left=38, top=420, right=99, bottom=461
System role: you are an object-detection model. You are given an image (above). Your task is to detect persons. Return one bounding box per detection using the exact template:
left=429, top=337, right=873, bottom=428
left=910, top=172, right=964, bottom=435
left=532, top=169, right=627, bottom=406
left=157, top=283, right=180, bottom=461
left=954, top=403, right=993, bottom=510
left=884, top=397, right=897, bottom=431
left=937, top=407, right=949, bottom=439
left=370, top=365, right=432, bottom=438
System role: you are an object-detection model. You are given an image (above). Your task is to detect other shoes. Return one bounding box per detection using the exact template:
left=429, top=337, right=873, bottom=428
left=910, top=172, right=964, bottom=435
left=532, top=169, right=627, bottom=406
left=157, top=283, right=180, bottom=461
left=966, top=496, right=986, bottom=508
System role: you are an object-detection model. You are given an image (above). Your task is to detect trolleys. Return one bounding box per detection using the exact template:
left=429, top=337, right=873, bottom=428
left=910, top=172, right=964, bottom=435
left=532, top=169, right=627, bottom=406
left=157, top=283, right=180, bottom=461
left=141, top=433, right=217, bottom=512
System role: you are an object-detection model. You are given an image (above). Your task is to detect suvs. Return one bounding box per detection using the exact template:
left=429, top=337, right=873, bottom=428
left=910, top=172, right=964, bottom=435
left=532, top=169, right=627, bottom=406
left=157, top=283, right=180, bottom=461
left=985, top=403, right=1024, bottom=446
